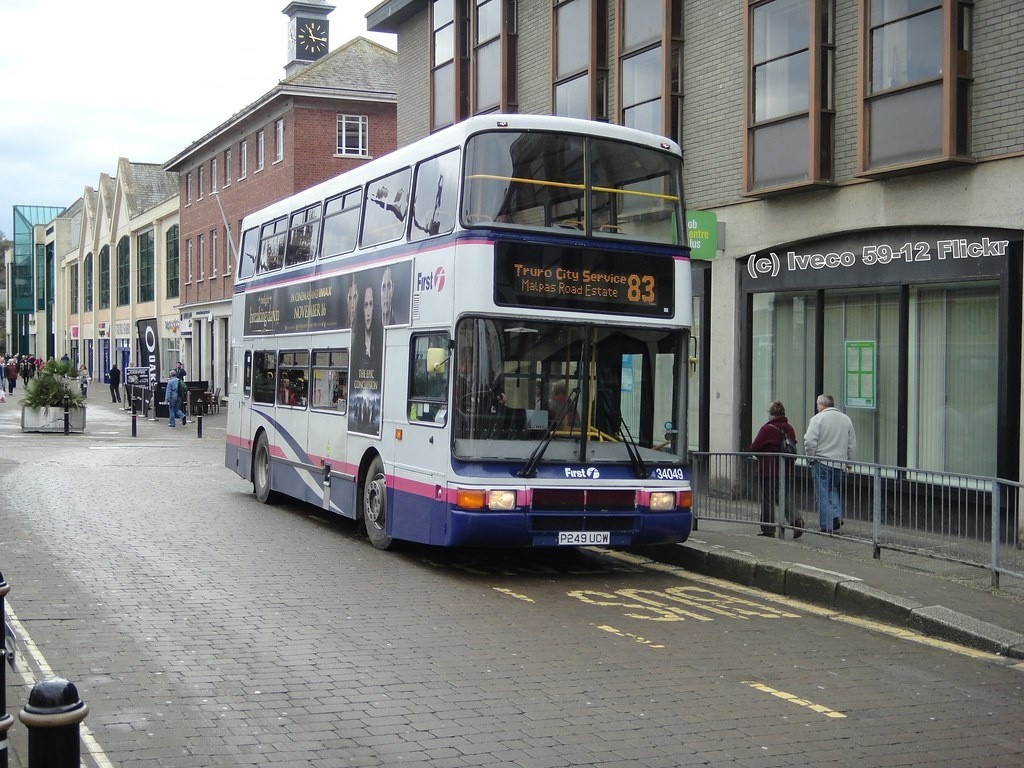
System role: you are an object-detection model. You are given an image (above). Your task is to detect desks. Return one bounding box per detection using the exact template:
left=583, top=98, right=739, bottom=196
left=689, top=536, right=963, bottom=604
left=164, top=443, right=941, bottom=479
left=185, top=392, right=213, bottom=414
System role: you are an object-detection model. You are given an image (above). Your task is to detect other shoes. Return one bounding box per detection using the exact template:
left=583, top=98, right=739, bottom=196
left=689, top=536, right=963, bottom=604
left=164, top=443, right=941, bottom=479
left=833, top=516, right=844, bottom=530
left=818, top=528, right=832, bottom=534
left=167, top=423, right=175, bottom=427
left=182, top=416, right=186, bottom=425
left=111, top=401, right=116, bottom=403
left=118, top=399, right=121, bottom=403
left=757, top=530, right=774, bottom=538
left=793, top=518, right=804, bottom=539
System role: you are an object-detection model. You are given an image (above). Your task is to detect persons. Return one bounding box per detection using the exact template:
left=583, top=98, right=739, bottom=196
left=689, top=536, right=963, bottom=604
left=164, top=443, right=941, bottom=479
left=61, top=353, right=70, bottom=380
left=80, top=365, right=89, bottom=400
left=531, top=378, right=582, bottom=430
left=750, top=402, right=805, bottom=539
left=344, top=265, right=395, bottom=435
left=445, top=344, right=508, bottom=410
left=164, top=362, right=190, bottom=427
left=803, top=394, right=857, bottom=534
left=0, top=353, right=54, bottom=403
left=109, top=363, right=121, bottom=403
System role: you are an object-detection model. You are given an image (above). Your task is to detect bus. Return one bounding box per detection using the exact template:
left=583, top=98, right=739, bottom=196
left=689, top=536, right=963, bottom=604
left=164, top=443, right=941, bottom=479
left=225, top=114, right=698, bottom=549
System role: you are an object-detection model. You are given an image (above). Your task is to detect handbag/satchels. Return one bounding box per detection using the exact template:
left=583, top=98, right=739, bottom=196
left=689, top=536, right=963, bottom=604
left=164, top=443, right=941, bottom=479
left=0, top=388, right=6, bottom=403
left=178, top=378, right=183, bottom=397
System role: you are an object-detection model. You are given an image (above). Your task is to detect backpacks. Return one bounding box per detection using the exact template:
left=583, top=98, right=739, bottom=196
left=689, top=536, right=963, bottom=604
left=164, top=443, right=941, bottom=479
left=768, top=422, right=798, bottom=464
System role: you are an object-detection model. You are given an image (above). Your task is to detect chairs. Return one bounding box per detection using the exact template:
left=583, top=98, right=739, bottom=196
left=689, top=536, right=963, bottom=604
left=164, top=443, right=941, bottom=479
left=212, top=388, right=221, bottom=413
left=424, top=211, right=520, bottom=235
left=255, top=369, right=310, bottom=407
left=190, top=389, right=208, bottom=415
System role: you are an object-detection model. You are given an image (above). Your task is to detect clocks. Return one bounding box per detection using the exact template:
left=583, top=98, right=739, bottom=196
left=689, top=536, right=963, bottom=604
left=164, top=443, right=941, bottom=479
left=296, top=17, right=330, bottom=60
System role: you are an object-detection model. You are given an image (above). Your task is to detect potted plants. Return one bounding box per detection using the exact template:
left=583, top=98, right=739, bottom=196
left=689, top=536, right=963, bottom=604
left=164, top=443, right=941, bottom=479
left=18, top=357, right=87, bottom=433
